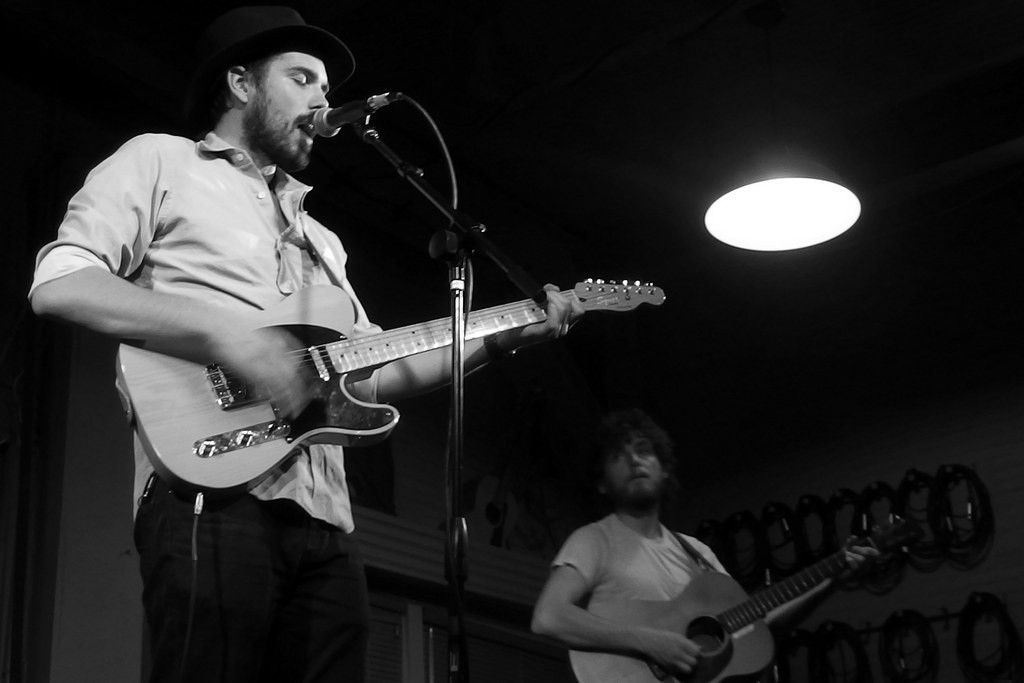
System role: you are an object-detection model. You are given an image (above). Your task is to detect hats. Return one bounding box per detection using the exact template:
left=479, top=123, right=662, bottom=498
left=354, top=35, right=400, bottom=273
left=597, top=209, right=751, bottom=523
left=184, top=6, right=355, bottom=106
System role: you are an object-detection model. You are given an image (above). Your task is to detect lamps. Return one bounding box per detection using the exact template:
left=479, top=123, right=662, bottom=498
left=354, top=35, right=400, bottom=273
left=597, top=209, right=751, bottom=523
left=703, top=1, right=863, bottom=254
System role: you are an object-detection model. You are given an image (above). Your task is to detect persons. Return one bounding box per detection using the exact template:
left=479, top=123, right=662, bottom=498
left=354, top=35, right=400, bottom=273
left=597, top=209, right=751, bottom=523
left=531, top=409, right=881, bottom=683
left=30, top=8, right=587, bottom=683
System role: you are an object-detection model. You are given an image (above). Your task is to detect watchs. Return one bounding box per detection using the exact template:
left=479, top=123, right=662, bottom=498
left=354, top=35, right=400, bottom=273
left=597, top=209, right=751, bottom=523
left=487, top=336, right=521, bottom=365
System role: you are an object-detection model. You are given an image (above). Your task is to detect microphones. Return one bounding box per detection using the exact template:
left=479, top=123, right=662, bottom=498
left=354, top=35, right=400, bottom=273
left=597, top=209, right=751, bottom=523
left=313, top=92, right=402, bottom=137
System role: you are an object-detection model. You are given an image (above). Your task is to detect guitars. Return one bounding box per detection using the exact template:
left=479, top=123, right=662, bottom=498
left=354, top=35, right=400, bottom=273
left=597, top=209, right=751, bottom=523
left=568, top=508, right=924, bottom=683
left=116, top=275, right=668, bottom=490
